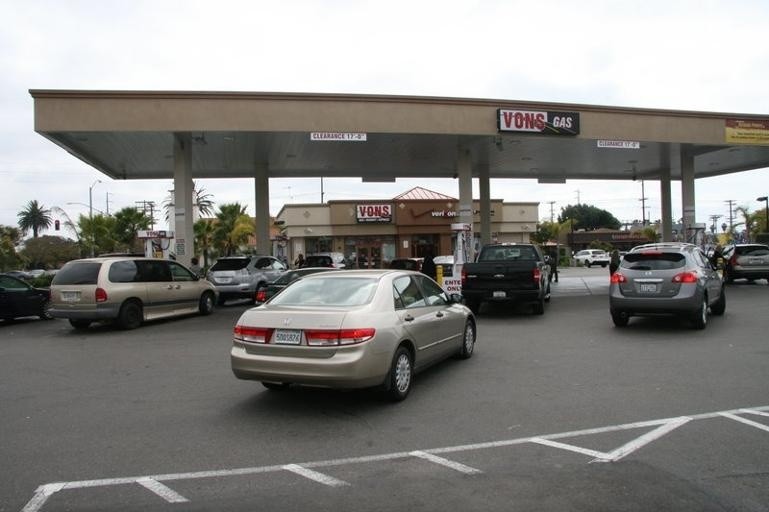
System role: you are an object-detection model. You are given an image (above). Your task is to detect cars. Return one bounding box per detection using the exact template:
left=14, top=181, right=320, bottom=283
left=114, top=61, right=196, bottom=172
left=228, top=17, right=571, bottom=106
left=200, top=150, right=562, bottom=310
left=0, top=269, right=54, bottom=319
left=574, top=249, right=609, bottom=268
left=230, top=256, right=470, bottom=401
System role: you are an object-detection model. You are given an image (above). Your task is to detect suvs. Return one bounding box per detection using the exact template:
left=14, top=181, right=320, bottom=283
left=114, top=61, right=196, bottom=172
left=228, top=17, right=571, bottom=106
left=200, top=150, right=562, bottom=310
left=609, top=242, right=725, bottom=329
left=206, top=255, right=287, bottom=307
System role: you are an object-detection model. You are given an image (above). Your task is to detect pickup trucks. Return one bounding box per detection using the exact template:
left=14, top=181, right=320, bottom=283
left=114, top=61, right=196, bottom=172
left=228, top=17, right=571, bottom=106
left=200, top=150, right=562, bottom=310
left=461, top=242, right=551, bottom=314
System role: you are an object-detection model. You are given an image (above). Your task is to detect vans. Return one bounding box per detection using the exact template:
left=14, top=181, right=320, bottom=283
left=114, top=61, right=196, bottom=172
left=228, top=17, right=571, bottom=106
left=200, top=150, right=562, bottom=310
left=50, top=257, right=220, bottom=330
left=722, top=244, right=769, bottom=283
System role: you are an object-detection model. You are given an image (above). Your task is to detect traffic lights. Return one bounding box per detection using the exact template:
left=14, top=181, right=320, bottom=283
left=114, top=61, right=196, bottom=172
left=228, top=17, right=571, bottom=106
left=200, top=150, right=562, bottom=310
left=55, top=221, right=59, bottom=230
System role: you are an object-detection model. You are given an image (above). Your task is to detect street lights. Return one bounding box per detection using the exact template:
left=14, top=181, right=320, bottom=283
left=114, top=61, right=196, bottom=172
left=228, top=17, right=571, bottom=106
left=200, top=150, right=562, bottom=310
left=90, top=179, right=101, bottom=219
left=757, top=197, right=769, bottom=232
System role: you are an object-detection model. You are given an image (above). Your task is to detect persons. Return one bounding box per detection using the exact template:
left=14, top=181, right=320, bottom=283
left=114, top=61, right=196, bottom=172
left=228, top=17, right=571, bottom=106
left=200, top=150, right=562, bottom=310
left=295, top=253, right=304, bottom=268
left=422, top=254, right=437, bottom=280
left=189, top=256, right=200, bottom=277
left=548, top=245, right=559, bottom=283
left=609, top=250, right=620, bottom=276
left=711, top=244, right=730, bottom=286
